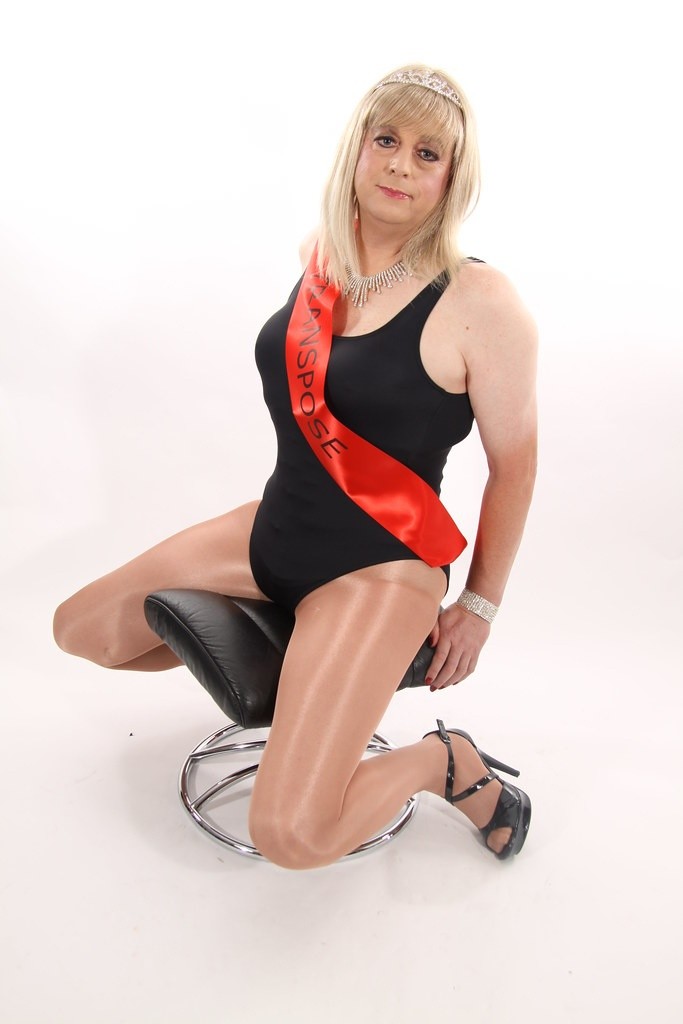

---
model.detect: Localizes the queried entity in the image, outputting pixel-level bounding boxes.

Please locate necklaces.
[341,260,413,307]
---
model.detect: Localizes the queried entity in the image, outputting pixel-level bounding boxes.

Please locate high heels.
[425,718,532,861]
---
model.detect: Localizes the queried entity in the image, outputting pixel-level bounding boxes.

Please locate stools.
[144,589,445,863]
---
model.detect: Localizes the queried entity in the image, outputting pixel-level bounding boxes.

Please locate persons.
[54,65,538,869]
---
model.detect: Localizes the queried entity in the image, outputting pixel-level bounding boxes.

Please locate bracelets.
[456,588,499,624]
